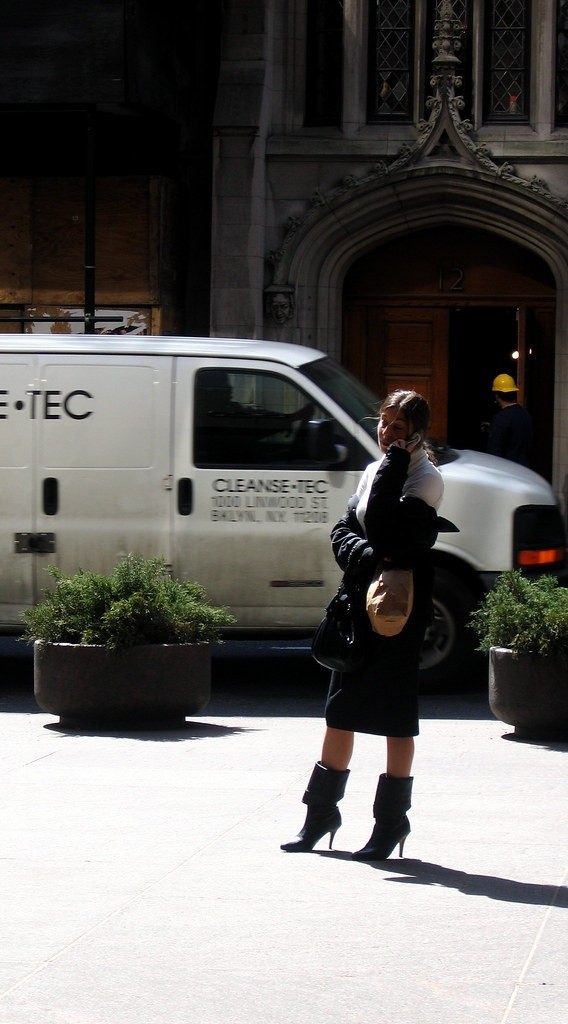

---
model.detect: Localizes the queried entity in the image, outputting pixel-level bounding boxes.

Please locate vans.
[0,335,568,691]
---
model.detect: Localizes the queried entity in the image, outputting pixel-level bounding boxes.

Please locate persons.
[489,373,534,469]
[268,293,290,325]
[281,387,459,862]
[196,369,314,464]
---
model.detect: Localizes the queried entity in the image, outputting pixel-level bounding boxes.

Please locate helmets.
[492,374,520,392]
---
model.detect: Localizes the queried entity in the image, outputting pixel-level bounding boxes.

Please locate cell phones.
[405,432,422,448]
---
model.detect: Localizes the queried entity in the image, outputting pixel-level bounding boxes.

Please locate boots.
[351,773,413,862]
[279,761,350,853]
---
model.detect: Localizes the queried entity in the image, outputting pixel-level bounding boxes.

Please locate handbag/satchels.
[310,538,383,676]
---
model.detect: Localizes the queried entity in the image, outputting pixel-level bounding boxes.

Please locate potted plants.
[14,549,238,732]
[464,568,568,739]
